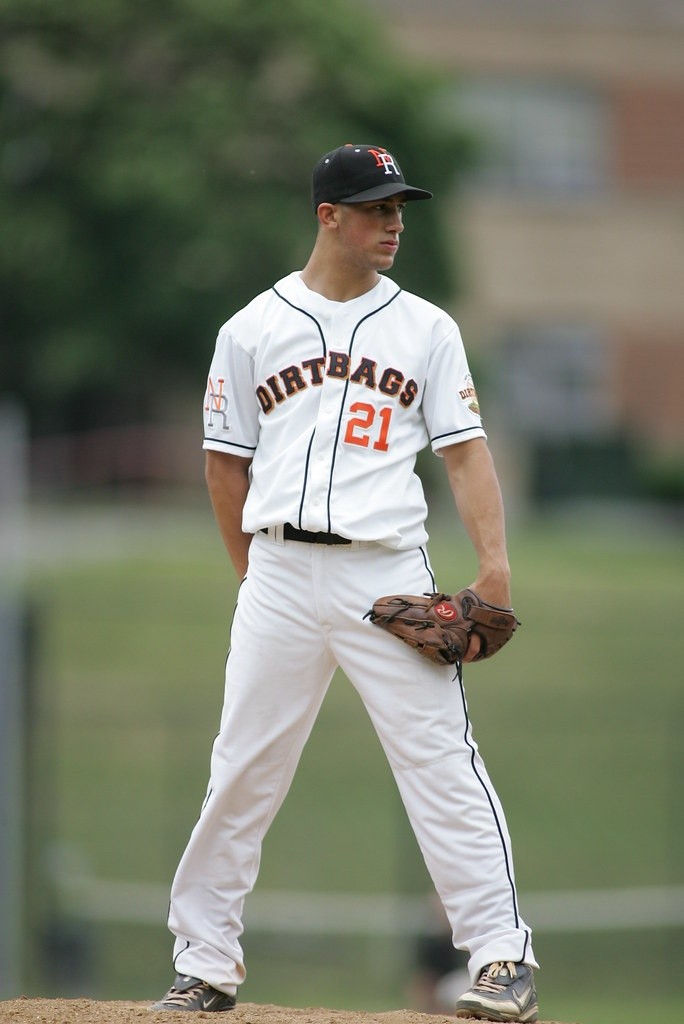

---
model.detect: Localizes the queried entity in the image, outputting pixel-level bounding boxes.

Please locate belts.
[259,524,352,545]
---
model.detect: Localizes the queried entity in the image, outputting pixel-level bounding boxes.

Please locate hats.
[312,144,433,217]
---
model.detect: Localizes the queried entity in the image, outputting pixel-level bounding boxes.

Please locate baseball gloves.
[369,588,518,666]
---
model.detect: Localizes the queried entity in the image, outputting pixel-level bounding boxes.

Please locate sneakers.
[456,961,538,1022]
[147,975,235,1010]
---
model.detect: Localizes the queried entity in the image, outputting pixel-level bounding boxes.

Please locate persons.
[158,140,542,1023]
